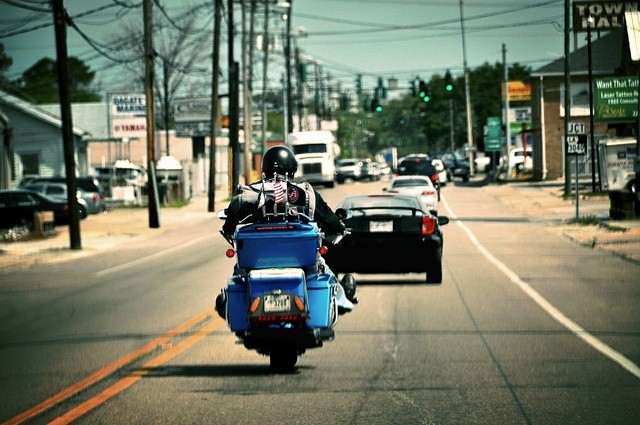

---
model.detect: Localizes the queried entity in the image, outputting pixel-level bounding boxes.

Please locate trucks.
[288,129,336,186]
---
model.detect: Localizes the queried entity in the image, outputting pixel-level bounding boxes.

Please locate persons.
[220,144,346,267]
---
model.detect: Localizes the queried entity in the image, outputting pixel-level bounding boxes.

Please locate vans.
[398,152,440,200]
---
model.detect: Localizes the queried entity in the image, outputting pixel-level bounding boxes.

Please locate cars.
[444,151,469,182]
[432,160,446,186]
[383,175,438,207]
[324,193,449,282]
[339,158,384,180]
[0,188,87,228]
[25,181,105,214]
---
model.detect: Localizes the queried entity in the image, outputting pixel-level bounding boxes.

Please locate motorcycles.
[216,206,358,371]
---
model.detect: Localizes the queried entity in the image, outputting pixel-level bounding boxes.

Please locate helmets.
[261,145,298,176]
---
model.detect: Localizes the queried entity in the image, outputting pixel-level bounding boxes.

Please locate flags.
[286,180,306,207]
[274,181,284,203]
[256,181,266,210]
